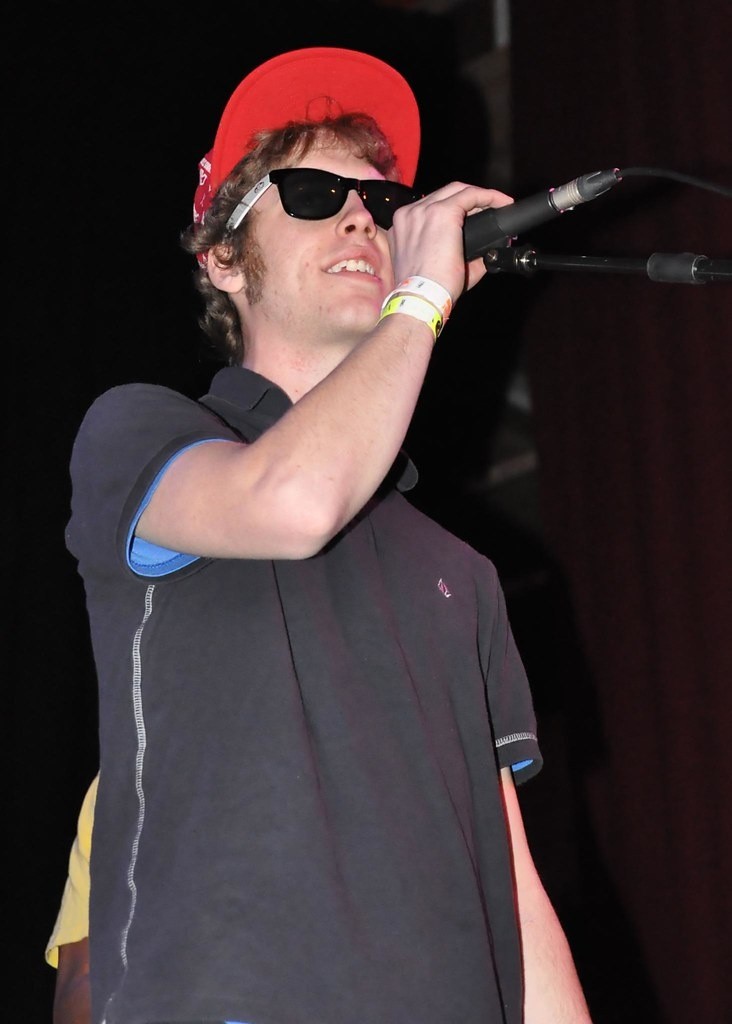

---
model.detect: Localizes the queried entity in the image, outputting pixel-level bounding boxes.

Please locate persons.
[43,768,102,1024]
[66,49,595,1024]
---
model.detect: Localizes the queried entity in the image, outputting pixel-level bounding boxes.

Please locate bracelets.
[379,274,452,337]
[375,295,444,342]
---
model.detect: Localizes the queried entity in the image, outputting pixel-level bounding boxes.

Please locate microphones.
[462,167,621,263]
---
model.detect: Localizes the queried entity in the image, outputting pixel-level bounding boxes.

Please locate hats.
[194,46,421,275]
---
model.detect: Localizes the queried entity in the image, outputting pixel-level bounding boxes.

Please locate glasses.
[223,167,425,244]
